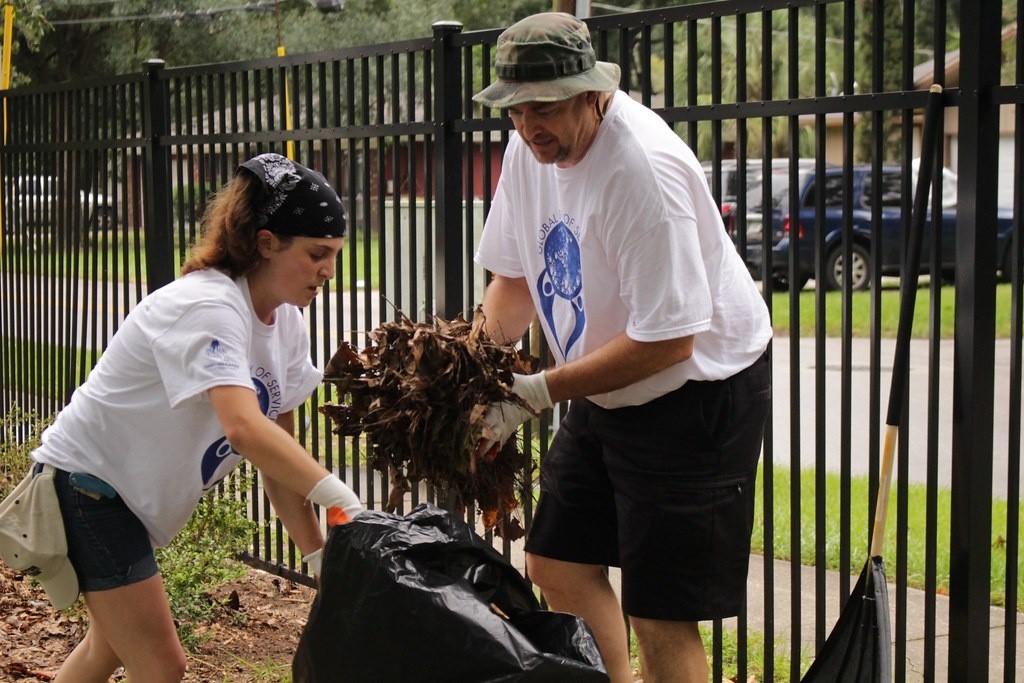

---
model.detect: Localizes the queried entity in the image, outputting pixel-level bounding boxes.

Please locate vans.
[701,159,831,198]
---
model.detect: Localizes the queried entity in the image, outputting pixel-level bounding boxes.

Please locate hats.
[0,461,78,609]
[39,461,39,462]
[472,12,621,108]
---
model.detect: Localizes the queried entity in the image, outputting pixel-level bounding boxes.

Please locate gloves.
[307,474,364,527]
[470,369,555,458]
[302,547,323,584]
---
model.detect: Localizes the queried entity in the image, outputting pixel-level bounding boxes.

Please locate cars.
[719,168,783,233]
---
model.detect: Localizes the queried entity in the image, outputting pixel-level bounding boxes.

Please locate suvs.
[4,177,115,230]
[746,167,1012,291]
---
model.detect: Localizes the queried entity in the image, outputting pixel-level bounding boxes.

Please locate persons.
[28,152,366,683]
[463,13,774,683]
